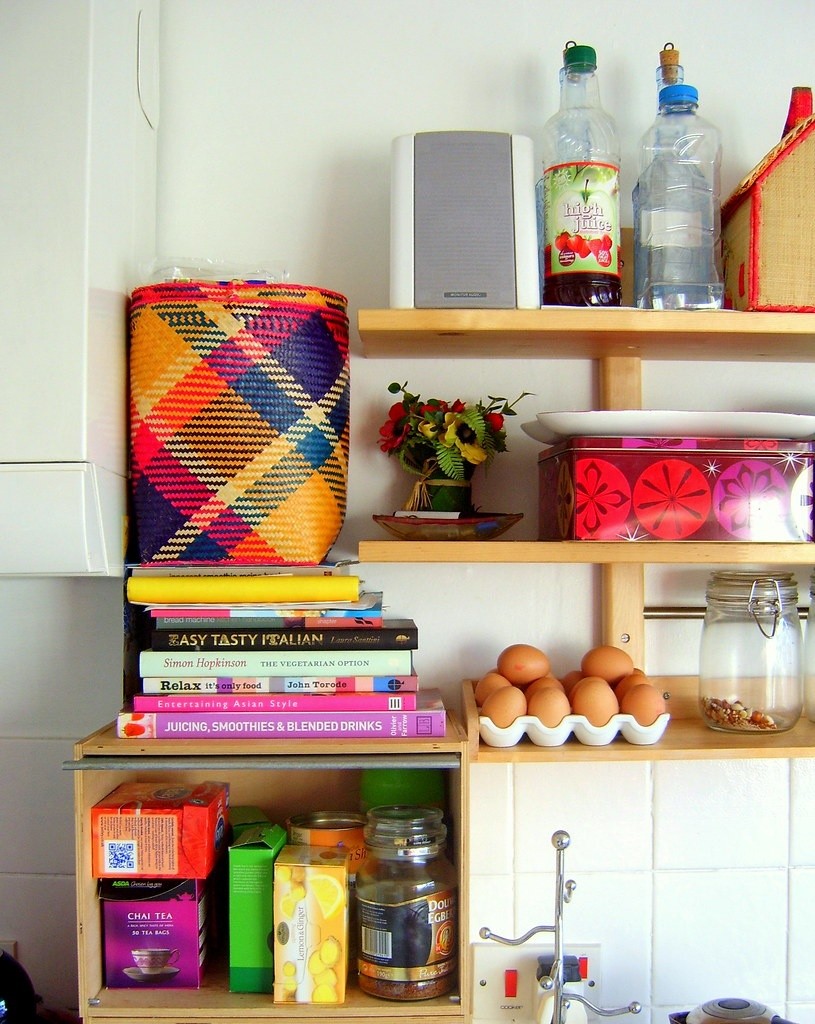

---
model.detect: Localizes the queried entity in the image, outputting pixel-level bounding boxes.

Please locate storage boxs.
[90,780,350,1005]
[537,437,815,544]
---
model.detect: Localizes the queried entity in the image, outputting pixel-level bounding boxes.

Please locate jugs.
[669,1002,792,1024]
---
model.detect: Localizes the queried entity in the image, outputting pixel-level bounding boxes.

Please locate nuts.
[700,697,777,731]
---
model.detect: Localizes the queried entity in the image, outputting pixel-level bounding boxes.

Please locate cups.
[134,947,179,973]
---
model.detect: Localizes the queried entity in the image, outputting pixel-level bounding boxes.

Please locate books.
[117,559,447,740]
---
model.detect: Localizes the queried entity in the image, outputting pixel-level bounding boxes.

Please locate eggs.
[475,644,668,728]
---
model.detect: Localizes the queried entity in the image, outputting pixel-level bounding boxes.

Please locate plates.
[124,968,180,984]
[519,405,815,446]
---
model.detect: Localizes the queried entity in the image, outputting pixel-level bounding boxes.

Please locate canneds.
[284,803,459,1000]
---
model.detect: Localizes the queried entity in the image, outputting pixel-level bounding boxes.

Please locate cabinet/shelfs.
[356,309,815,764]
[71,715,475,1024]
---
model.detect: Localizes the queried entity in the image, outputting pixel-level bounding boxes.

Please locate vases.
[407,444,476,514]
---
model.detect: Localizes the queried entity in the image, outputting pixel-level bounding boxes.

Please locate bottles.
[803,574,814,722]
[352,805,459,1001]
[699,569,802,735]
[533,43,727,310]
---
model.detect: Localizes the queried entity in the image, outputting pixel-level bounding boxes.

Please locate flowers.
[374,379,535,484]
